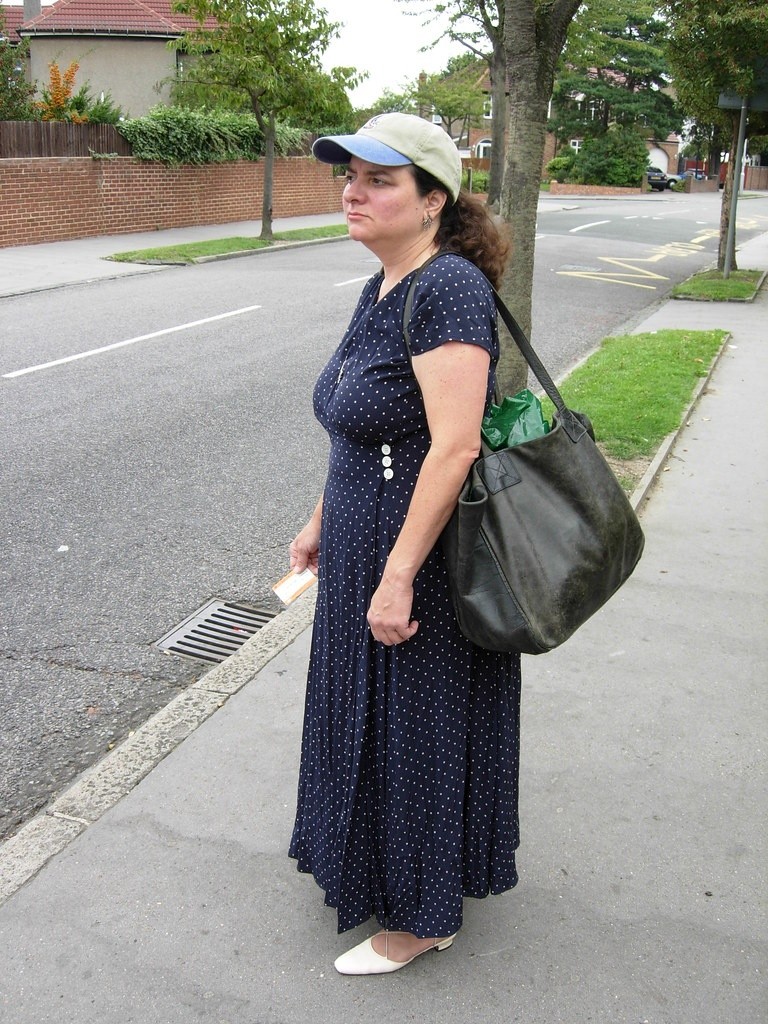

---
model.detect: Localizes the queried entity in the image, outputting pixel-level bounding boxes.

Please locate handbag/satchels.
[403,252,646,655]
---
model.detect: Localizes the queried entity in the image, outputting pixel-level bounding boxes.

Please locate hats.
[312,113,462,206]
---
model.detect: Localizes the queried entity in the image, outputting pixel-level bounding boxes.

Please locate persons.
[288,112,522,975]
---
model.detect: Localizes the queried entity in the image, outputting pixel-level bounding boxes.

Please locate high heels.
[334,928,457,975]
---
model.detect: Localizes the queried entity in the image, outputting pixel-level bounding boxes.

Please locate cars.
[665,174,681,190]
[646,167,667,191]
